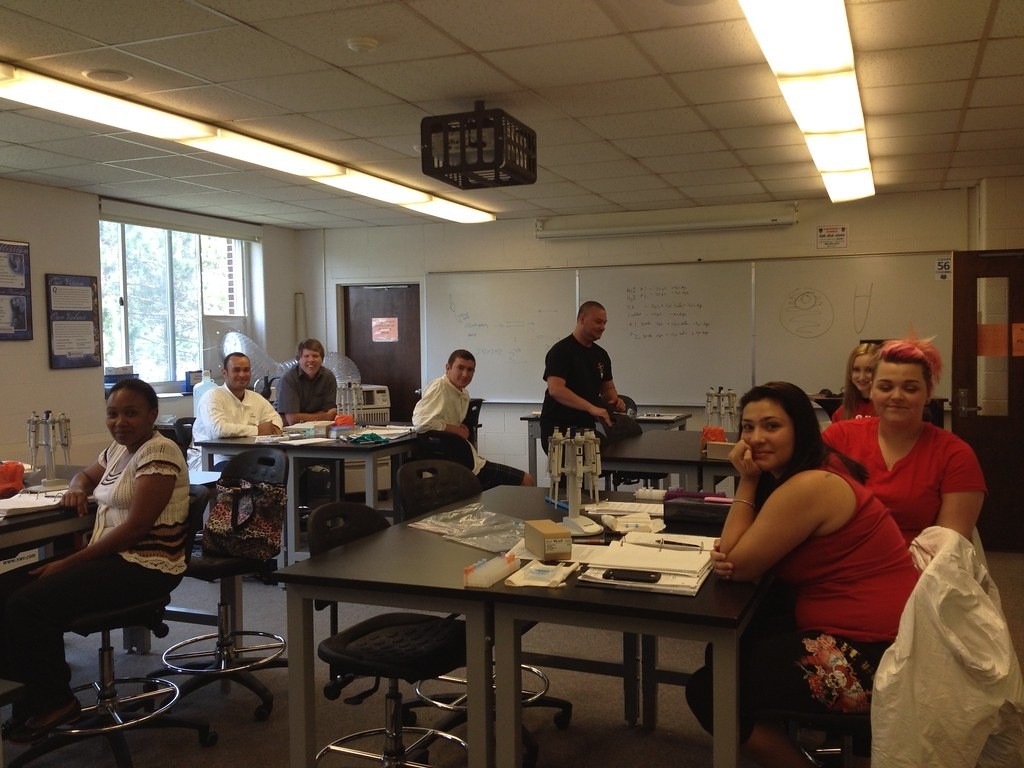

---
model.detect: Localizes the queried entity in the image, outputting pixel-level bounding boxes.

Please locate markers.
[644,414,660,417]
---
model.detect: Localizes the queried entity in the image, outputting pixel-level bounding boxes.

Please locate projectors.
[432,128,525,164]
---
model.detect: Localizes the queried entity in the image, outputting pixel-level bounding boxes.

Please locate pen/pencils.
[656,540,700,548]
[704,496,733,503]
[270,435,284,437]
[574,539,612,545]
[545,496,569,510]
[45,495,63,498]
[362,424,387,427]
[360,441,382,444]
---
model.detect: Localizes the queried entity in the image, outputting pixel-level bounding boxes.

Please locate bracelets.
[732,499,756,509]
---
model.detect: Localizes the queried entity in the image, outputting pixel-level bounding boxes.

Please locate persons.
[824,339,987,547]
[412,349,532,488]
[685,381,918,768]
[539,301,626,487]
[187,352,283,529]
[0,379,190,744]
[272,338,337,426]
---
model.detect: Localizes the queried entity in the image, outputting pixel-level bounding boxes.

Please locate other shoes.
[8,697,82,746]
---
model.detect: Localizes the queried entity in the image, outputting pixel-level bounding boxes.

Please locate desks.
[144,447,290,709]
[808,391,949,430]
[193,421,421,576]
[520,402,692,490]
[0,466,243,661]
[265,484,776,768]
[599,429,740,495]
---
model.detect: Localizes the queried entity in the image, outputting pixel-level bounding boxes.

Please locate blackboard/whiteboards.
[424,250,953,411]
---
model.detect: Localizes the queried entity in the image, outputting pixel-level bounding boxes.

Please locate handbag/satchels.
[202,479,286,560]
[601,414,642,444]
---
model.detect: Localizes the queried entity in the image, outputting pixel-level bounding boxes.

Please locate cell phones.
[705,496,733,504]
[603,568,662,584]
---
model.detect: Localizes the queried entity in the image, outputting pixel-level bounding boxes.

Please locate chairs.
[318,614,571,768]
[46,485,219,768]
